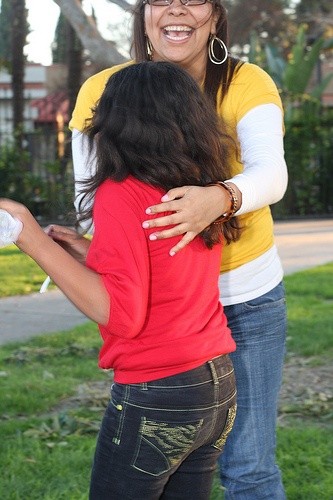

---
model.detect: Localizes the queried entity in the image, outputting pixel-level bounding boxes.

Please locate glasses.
[142,0,211,7]
